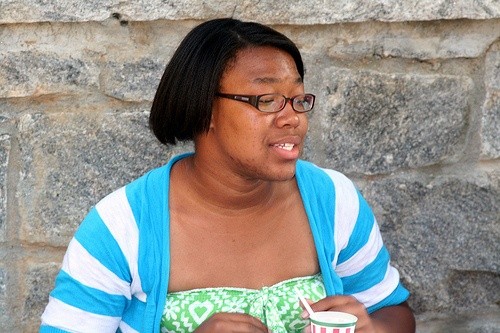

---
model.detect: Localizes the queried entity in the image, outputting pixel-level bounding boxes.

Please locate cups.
[309,311,358,333]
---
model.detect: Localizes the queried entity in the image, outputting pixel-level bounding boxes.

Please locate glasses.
[215,92,316,113]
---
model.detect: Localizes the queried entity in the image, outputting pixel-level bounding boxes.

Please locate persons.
[38,18,416,333]
